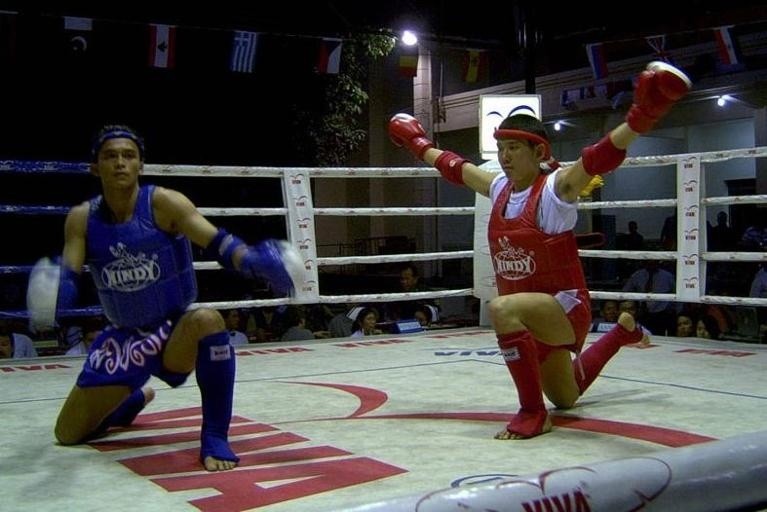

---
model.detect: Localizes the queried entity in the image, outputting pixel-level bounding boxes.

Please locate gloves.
[623,60,693,133]
[239,239,306,298]
[25,258,80,336]
[387,112,434,162]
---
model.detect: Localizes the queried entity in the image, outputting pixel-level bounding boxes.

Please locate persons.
[219,264,458,344]
[25,124,306,472]
[387,56,694,441]
[595,208,767,340]
[1,316,110,358]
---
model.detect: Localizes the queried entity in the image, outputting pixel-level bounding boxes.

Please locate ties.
[645,272,652,293]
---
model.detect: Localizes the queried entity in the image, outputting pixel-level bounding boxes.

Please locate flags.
[458,48,489,85]
[646,36,676,66]
[585,41,608,81]
[227,29,257,74]
[398,45,419,78]
[712,27,745,67]
[146,22,178,70]
[61,17,92,56]
[312,38,343,75]
